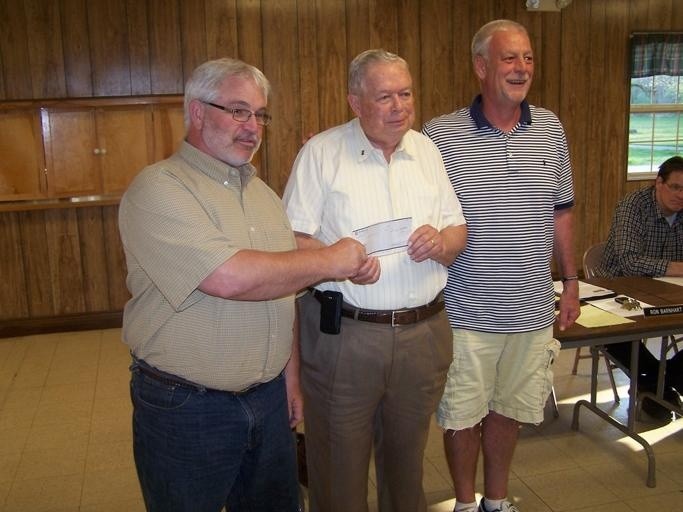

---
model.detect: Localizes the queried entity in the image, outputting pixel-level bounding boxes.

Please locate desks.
[549,276,682,488]
[617,277,682,424]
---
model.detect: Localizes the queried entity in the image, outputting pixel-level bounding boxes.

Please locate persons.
[418,15,583,512]
[589,153,683,419]
[280,48,470,512]
[118,58,366,512]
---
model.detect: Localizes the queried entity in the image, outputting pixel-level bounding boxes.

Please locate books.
[550,280,614,300]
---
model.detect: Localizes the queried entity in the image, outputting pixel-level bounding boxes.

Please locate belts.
[306,287,445,327]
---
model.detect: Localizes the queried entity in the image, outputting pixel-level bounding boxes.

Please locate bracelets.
[560,276,578,280]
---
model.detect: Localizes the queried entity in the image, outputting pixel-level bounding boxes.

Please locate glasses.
[209,102,272,127]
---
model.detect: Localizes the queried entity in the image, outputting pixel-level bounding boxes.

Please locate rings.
[429,239,437,246]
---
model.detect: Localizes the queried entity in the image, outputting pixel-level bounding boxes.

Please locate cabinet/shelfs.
[153,94,256,170]
[0,100,47,213]
[36,95,153,211]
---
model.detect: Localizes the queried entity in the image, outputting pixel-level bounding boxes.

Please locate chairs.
[572,237,680,406]
